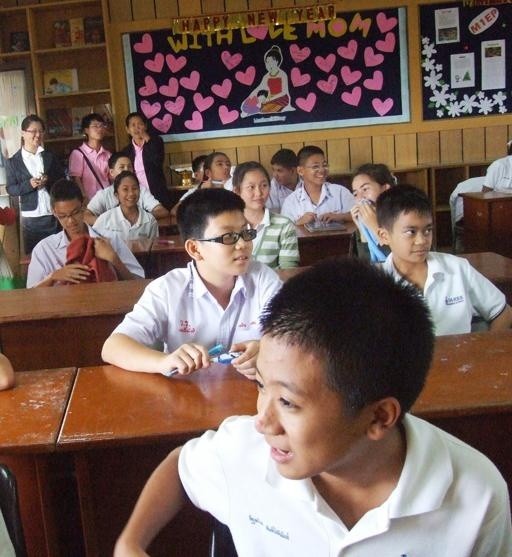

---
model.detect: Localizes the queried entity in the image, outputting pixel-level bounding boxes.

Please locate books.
[8,29,31,52]
[84,15,106,45]
[42,67,80,96]
[69,17,86,47]
[52,20,72,48]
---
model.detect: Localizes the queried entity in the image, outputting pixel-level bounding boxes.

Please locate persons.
[6,113,172,289]
[351,162,395,262]
[375,184,511,337]
[102,358,260,427]
[0,345,18,397]
[113,257,512,557]
[171,146,356,270]
[99,186,289,383]
[480,141,512,194]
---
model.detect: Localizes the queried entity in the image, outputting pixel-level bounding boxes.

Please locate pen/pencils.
[166,344,224,378]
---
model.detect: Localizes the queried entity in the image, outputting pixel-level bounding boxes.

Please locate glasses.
[300,164,329,170]
[191,228,258,246]
[24,128,45,134]
[90,122,107,128]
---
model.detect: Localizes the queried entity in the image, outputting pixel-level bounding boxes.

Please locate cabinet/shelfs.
[0,1,130,159]
[328,163,492,252]
